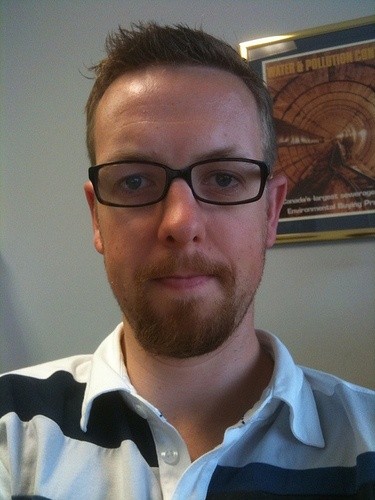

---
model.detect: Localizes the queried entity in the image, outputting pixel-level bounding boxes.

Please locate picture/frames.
[239,15,375,244]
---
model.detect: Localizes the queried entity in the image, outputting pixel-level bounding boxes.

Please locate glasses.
[87,157,274,207]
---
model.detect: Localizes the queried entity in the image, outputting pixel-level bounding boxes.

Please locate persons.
[0,21,375,500]
